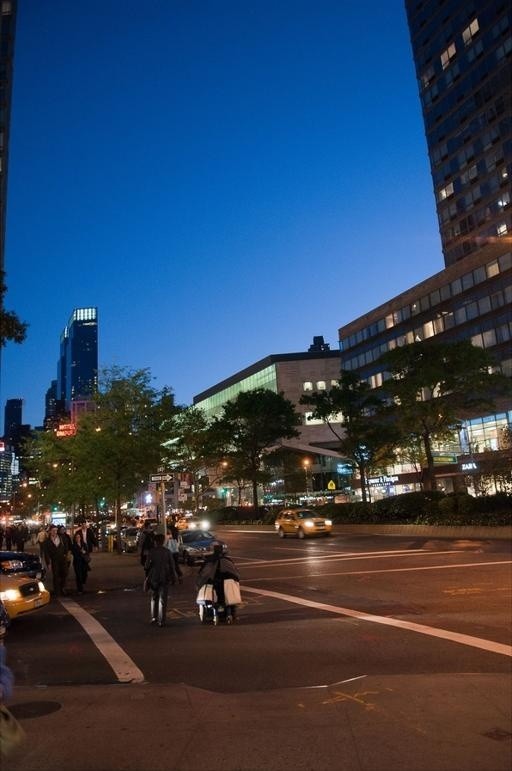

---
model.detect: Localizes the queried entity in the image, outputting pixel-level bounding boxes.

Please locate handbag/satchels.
[80,553,91,563]
[169,559,178,582]
[223,578,242,607]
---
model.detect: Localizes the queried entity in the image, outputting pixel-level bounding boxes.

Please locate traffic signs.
[149,472,175,482]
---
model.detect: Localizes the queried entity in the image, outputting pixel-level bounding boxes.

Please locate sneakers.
[148,618,164,628]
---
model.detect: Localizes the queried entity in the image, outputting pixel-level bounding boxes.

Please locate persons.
[0,600,14,720]
[165,525,184,585]
[198,544,234,572]
[144,533,181,628]
[138,521,156,564]
[0,518,99,596]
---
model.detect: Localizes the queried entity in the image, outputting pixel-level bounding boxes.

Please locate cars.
[0,573,50,619]
[274,508,334,538]
[91,516,229,566]
[0,549,47,581]
